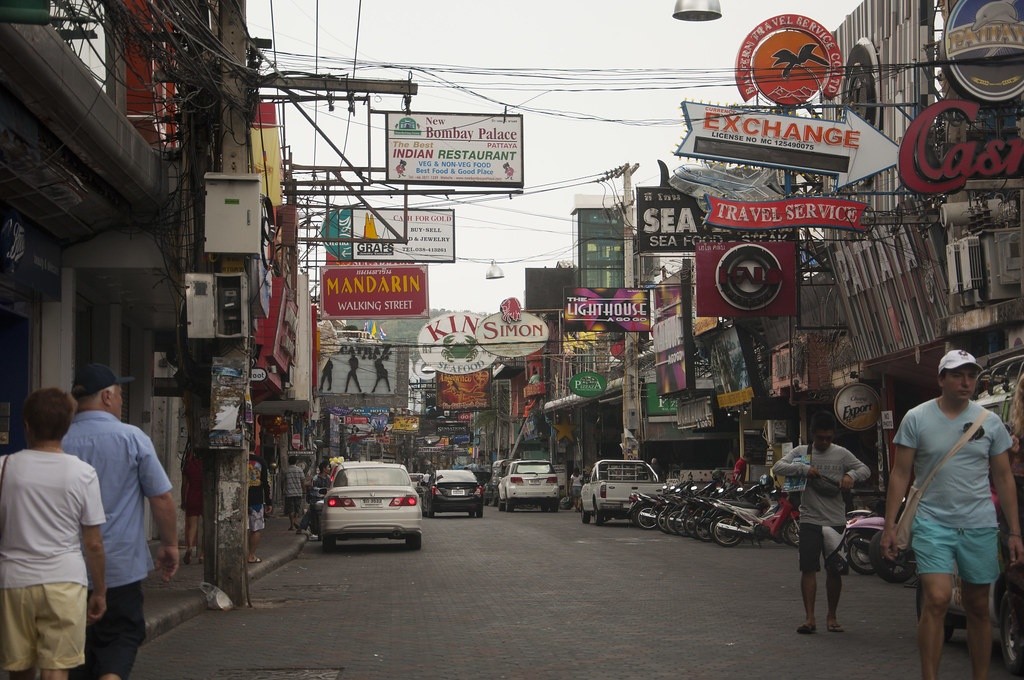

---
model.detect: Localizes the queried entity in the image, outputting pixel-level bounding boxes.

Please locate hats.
[71,363,135,399]
[938,349,983,376]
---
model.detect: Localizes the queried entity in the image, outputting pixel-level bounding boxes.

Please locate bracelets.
[1009,533,1023,538]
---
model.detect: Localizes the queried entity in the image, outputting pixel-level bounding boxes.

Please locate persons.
[0,389,108,680]
[880,350,1024,680]
[178,434,330,568]
[772,412,872,633]
[732,450,753,486]
[993,373,1024,538]
[57,363,180,680]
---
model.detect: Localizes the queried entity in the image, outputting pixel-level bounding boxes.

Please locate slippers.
[797,623,816,633]
[827,625,843,632]
[247,558,262,563]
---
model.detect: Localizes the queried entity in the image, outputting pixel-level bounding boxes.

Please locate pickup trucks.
[580,459,667,526]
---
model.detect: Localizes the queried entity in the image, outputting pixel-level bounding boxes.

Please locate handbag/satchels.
[807,474,841,498]
[895,485,921,550]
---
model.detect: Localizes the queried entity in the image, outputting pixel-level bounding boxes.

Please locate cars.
[311,458,560,552]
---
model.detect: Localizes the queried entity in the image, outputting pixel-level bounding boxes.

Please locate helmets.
[760,473,774,489]
[711,469,723,480]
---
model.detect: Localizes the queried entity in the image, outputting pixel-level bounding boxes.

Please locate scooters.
[627,468,885,575]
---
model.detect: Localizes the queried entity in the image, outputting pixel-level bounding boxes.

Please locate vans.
[914,354,1024,680]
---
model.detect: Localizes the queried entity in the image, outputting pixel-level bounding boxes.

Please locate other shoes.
[183,548,192,564]
[296,529,302,534]
[199,557,204,564]
